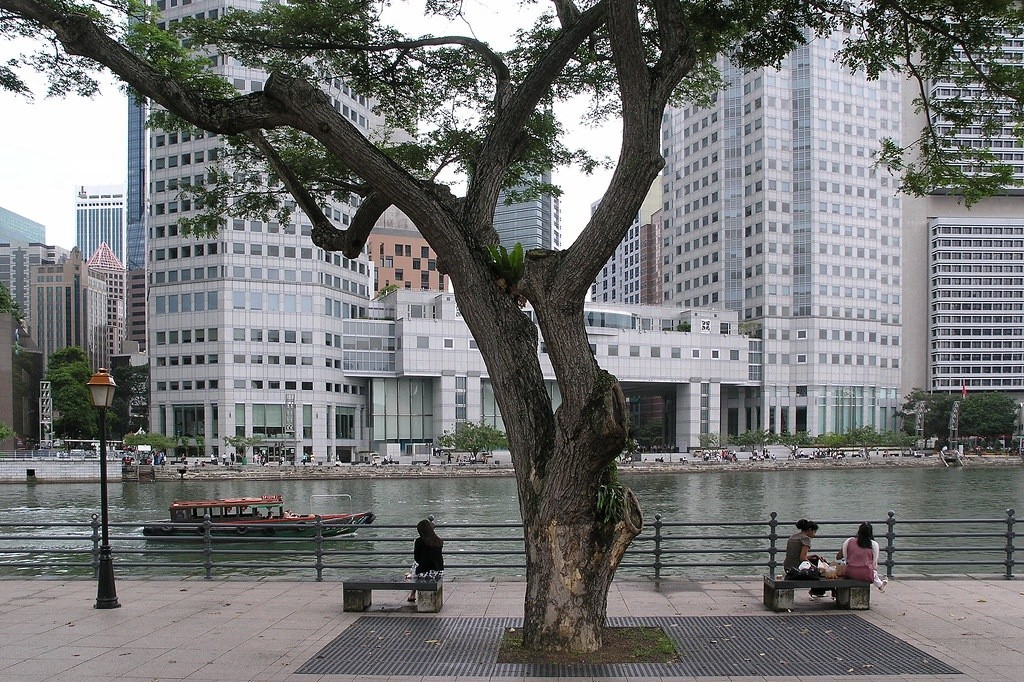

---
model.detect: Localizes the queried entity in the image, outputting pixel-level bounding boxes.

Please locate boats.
[144,495,376,537]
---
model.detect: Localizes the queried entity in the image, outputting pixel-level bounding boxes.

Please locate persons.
[222,453,227,465]
[311,453,316,465]
[383,455,393,463]
[470,455,475,464]
[211,452,215,464]
[252,452,266,466]
[843,521,888,590]
[407,520,444,601]
[181,453,186,461]
[784,519,836,600]
[336,454,339,461]
[257,509,301,520]
[682,445,889,464]
[279,457,284,465]
[481,455,487,464]
[114,444,167,465]
[303,453,308,465]
[231,454,235,465]
[448,451,452,463]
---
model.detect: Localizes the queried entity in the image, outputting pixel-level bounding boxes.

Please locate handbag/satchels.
[785,567,822,581]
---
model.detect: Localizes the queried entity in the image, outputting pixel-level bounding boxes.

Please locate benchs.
[342,574,444,612]
[763,574,870,611]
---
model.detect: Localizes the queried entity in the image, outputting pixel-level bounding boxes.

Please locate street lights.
[426,441,436,467]
[87,368,122,608]
[667,444,675,466]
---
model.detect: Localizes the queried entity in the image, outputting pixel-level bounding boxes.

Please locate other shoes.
[406,596,416,603]
[878,580,888,590]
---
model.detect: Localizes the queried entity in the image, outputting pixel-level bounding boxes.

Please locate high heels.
[808,589,826,598]
